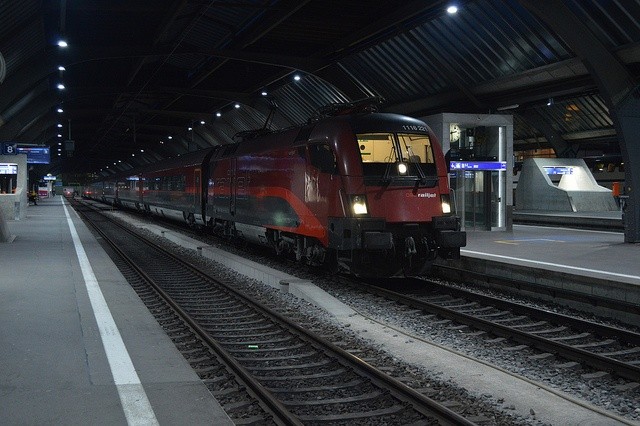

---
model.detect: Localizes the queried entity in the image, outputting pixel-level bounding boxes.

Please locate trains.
[79,95,466,281]
